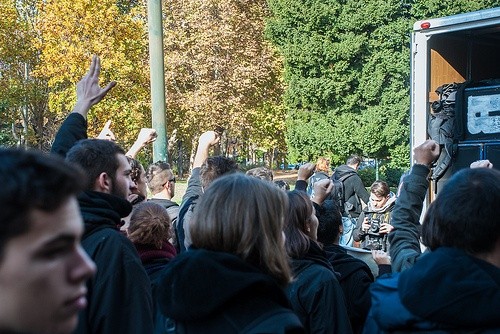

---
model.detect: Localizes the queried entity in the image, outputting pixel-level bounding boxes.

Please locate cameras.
[367,219,384,237]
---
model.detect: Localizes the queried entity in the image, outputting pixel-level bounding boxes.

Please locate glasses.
[162,176,176,187]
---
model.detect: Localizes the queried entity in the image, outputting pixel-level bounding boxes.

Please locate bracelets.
[417,163,429,167]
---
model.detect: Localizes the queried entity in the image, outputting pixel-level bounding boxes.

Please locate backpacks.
[334,171,357,214]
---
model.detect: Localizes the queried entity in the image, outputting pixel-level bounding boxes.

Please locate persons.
[0,54,500,334]
[331,155,369,246]
[353,180,397,251]
